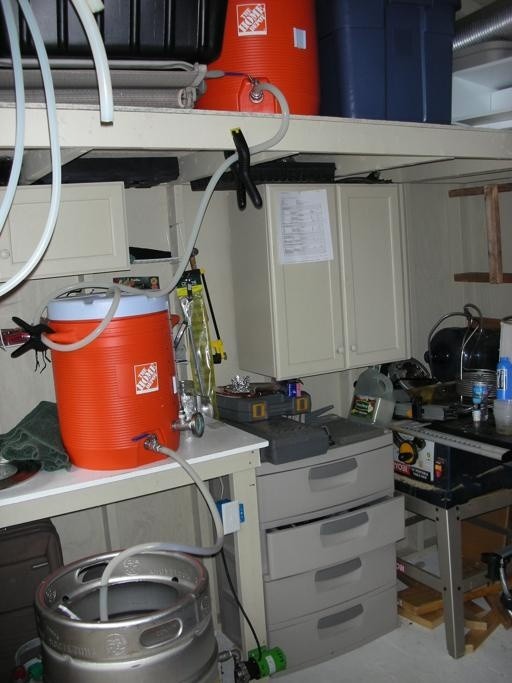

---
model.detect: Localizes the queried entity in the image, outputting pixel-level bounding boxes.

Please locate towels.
[0,400,72,472]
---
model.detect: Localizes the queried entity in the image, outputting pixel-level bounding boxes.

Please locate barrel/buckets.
[348,367,397,425]
[35,548,221,683]
[47,294,179,470]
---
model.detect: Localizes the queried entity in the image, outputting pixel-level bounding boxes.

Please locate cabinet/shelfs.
[209,415,406,679]
[0,182,130,283]
[228,182,411,383]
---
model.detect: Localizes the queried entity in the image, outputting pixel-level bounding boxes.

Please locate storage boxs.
[315,0,461,126]
[0,0,228,64]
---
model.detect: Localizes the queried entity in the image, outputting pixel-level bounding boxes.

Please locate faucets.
[132,433,160,453]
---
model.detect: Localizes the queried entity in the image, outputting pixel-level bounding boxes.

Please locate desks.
[377,421,511,662]
[0,416,269,662]
